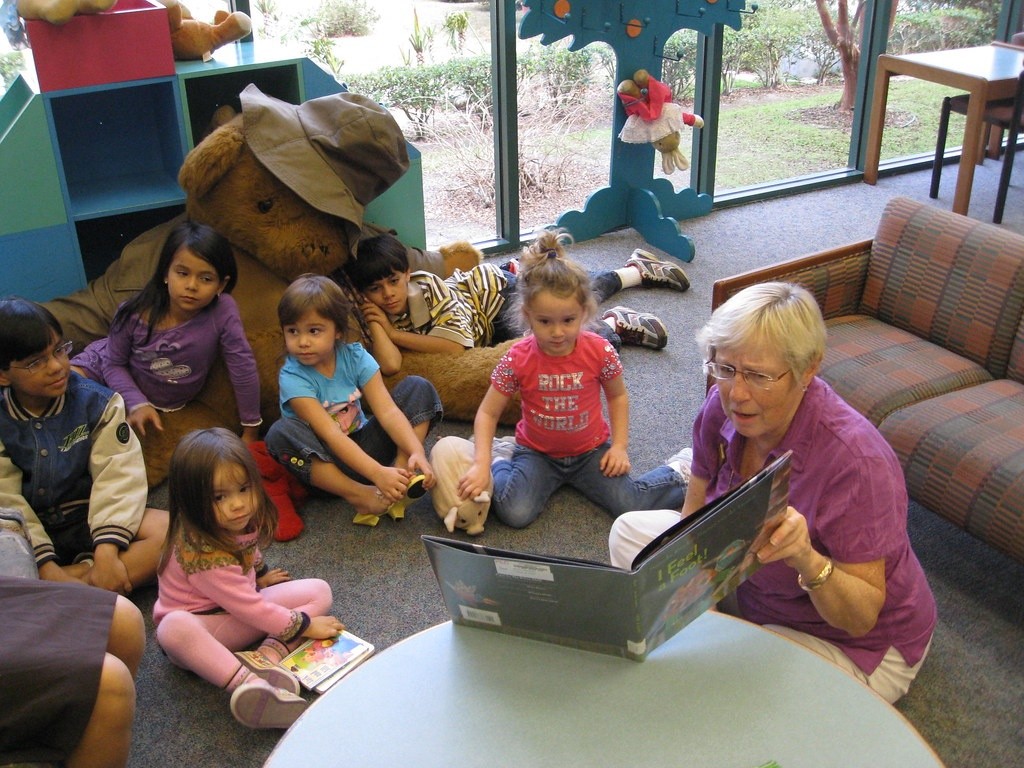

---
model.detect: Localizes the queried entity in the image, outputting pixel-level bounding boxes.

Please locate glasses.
[702,354,788,390]
[0,338,73,375]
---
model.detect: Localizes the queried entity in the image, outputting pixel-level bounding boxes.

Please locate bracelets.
[798,556,834,592]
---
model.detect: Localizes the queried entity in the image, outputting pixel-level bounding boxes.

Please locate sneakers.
[624,249,688,294]
[669,448,696,475]
[469,433,517,461]
[602,304,667,349]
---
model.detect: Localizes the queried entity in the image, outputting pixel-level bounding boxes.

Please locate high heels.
[386,472,431,523]
[352,498,399,527]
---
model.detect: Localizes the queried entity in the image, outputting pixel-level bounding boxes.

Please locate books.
[277,627,375,695]
[418,447,797,663]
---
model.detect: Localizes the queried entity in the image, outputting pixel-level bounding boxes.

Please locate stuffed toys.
[0,0,252,61]
[613,69,705,176]
[36,83,535,482]
[430,435,493,536]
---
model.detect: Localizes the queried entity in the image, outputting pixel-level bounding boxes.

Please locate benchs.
[705,197,1023,562]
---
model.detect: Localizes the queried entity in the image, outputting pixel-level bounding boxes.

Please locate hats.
[238,82,415,240]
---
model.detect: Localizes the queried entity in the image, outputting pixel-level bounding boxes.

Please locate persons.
[456,226,693,528]
[0,572,147,768]
[153,426,345,732]
[605,282,935,708]
[66,221,263,442]
[0,295,169,595]
[351,234,690,375]
[265,272,444,527]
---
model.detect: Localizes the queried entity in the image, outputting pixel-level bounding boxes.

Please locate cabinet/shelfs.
[0,1,426,299]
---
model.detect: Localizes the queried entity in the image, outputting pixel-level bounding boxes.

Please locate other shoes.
[235,650,301,697]
[231,681,306,729]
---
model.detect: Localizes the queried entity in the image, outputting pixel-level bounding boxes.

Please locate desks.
[863,42,1024,216]
[264,611,948,768]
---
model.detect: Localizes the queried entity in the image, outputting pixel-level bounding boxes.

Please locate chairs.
[929,59,1024,224]
